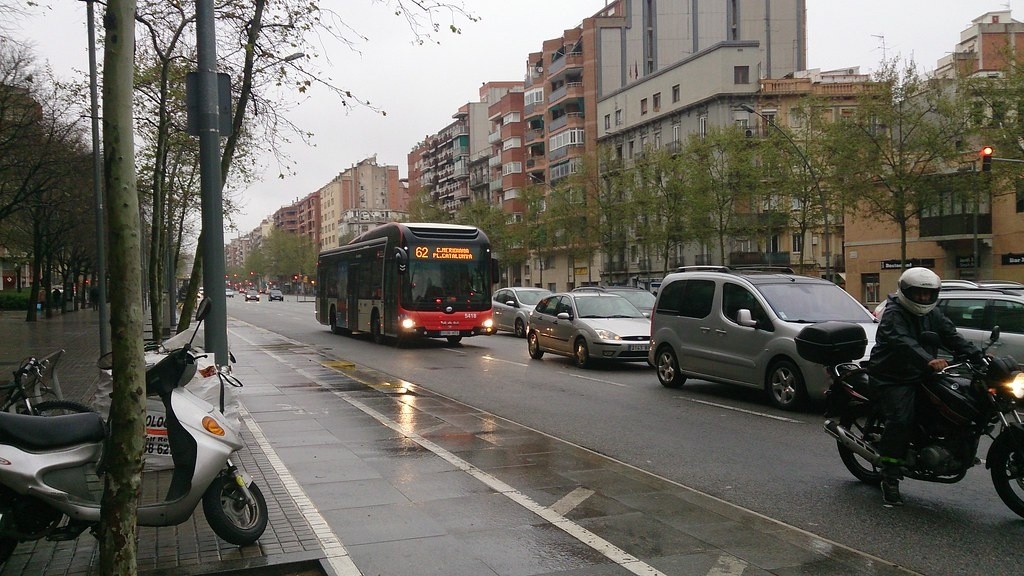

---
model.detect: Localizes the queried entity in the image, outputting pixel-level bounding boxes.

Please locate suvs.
[645,265,883,413]
[872,279,1024,370]
[569,285,656,321]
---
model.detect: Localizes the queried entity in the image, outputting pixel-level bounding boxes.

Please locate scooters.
[0,292,270,563]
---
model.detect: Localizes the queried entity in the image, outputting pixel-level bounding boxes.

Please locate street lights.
[740,103,832,281]
[523,170,592,285]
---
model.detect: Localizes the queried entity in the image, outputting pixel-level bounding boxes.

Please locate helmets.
[896,267,941,316]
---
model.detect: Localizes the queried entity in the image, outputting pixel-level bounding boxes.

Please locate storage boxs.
[794,321,868,366]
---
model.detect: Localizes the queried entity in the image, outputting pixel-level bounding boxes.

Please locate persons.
[54,288,61,313]
[457,278,472,295]
[90,284,99,311]
[869,268,995,508]
[40,290,45,313]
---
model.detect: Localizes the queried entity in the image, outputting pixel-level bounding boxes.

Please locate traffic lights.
[982,147,993,173]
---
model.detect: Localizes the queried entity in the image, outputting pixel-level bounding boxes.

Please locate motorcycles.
[822,325,1024,520]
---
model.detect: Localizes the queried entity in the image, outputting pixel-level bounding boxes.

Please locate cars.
[491,287,555,338]
[199,287,204,295]
[245,290,260,301]
[226,288,234,298]
[239,288,245,294]
[527,292,652,368]
[268,290,283,301]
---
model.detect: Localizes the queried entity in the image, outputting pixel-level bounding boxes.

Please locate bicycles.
[0,354,95,417]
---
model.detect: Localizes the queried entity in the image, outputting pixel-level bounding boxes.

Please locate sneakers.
[973,457,980,464]
[881,480,904,505]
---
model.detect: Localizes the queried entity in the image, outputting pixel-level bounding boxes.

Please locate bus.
[314,221,500,347]
